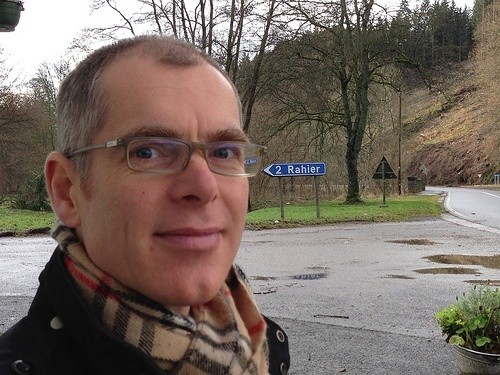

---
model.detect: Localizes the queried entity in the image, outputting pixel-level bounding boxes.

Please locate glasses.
[66,136,268,178]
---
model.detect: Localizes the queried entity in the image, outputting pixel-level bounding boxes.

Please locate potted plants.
[436,279,500,375]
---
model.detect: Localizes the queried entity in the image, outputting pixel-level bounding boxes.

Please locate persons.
[0,34,290,375]
